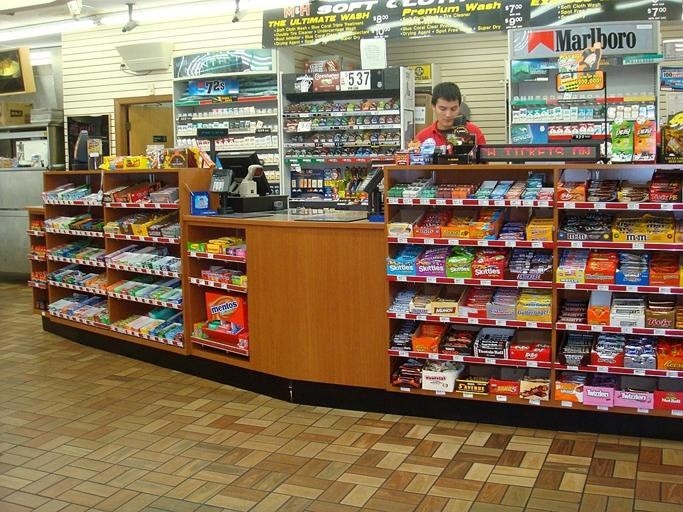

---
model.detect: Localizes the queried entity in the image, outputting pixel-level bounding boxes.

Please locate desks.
[183,210,387,391]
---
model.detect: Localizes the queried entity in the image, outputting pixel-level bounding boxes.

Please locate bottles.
[338,166,367,198]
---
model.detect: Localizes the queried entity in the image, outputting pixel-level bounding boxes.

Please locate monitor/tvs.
[217,152,273,195]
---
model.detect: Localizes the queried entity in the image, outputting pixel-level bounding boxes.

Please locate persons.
[411,82,486,147]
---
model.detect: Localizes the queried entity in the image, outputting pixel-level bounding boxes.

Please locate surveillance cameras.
[232,11,245,22]
[121,21,138,32]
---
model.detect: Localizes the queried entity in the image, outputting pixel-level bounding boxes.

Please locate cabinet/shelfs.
[382,164,683,420]
[279,67,415,213]
[23,168,219,356]
[507,18,662,164]
[170,45,295,196]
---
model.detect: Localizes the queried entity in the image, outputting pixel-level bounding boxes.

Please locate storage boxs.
[413,94,432,136]
